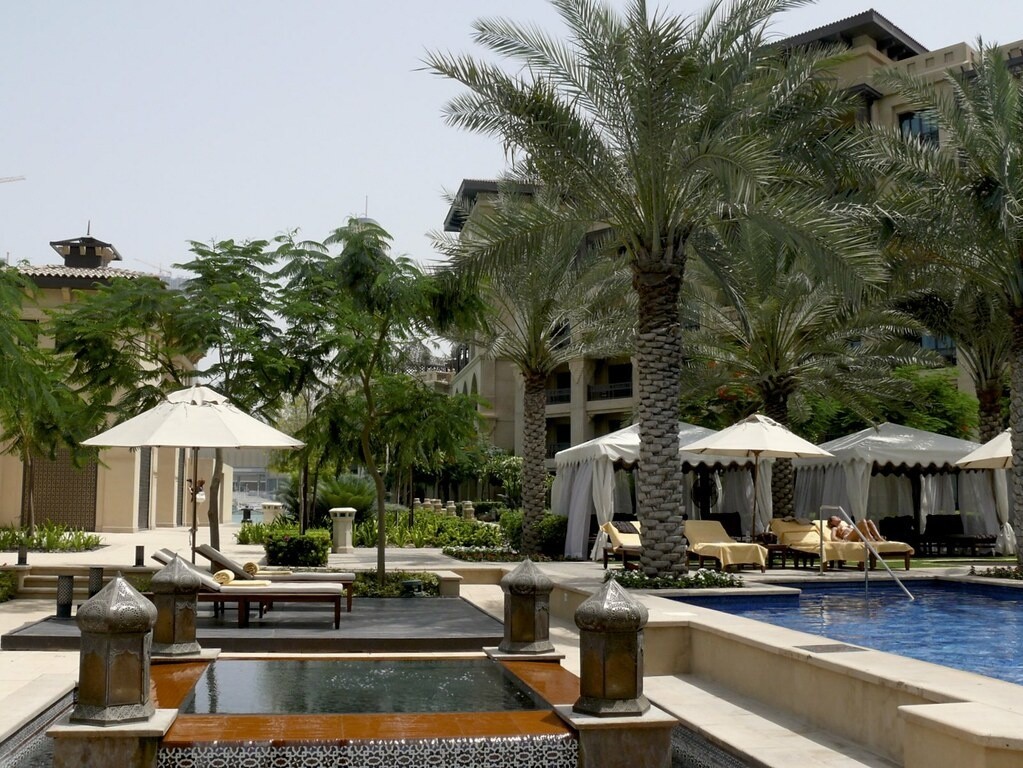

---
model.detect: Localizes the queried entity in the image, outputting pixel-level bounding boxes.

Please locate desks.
[761,544,789,568]
[213,601,267,618]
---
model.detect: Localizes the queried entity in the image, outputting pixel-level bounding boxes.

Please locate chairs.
[589,513,637,547]
[879,515,929,558]
[812,519,914,570]
[683,520,768,573]
[191,545,356,613]
[599,521,688,570]
[707,513,746,543]
[150,547,343,630]
[923,512,998,558]
[767,517,870,572]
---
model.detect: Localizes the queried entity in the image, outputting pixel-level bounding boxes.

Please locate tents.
[551,421,776,559]
[793,423,1016,556]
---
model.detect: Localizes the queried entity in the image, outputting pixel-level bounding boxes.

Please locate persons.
[828,515,886,543]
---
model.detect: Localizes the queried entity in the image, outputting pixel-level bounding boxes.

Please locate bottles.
[746,529,750,542]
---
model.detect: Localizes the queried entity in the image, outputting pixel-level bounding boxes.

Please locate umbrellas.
[679,410,836,541]
[79,384,307,565]
[955,426,1013,469]
